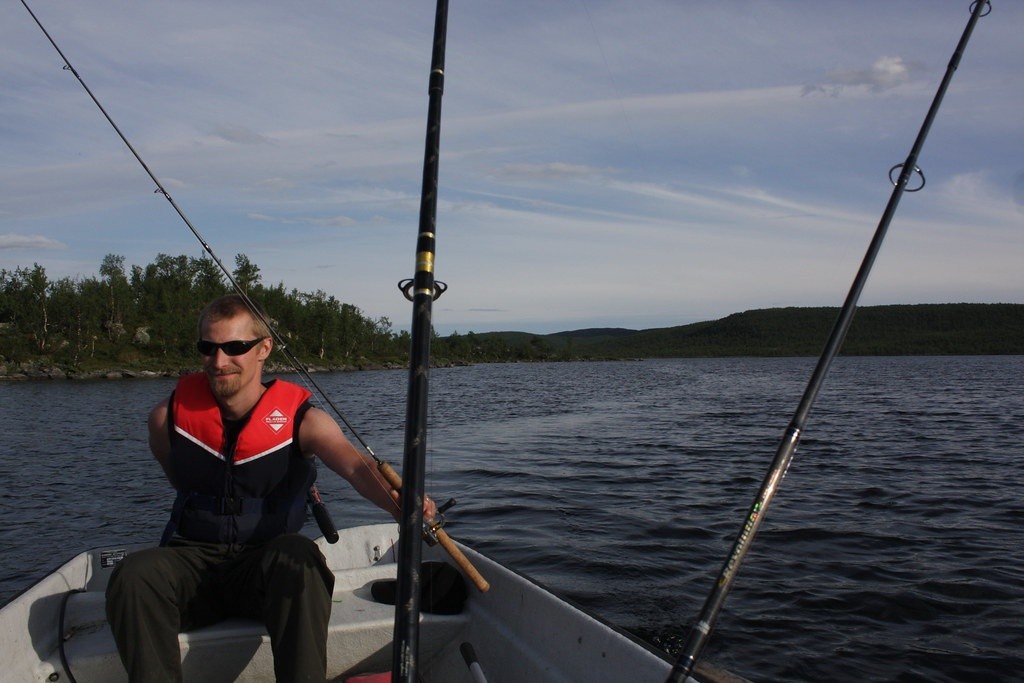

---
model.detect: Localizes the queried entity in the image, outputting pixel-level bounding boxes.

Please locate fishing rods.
[391,0,452,683]
[20,0,494,595]
[664,0,992,683]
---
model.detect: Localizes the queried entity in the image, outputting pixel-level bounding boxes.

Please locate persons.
[104,294,438,683]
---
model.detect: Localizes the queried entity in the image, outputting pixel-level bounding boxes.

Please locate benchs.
[58,563,471,683]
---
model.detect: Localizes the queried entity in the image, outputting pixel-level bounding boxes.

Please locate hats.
[371,561,467,615]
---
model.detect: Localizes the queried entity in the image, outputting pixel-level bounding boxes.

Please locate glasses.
[197,337,265,356]
